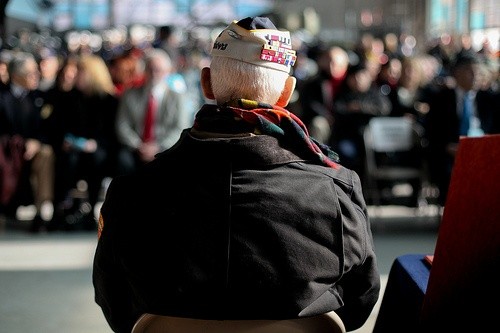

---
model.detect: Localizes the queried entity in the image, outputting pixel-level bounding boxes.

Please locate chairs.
[371,134,500,333]
[363,116,429,208]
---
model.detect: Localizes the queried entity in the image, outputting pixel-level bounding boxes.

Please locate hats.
[211,16,296,73]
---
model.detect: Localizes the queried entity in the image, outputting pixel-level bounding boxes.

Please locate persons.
[92,17,381,333]
[0,23,210,230]
[289,25,500,209]
